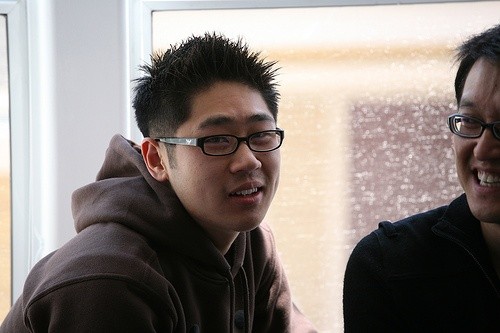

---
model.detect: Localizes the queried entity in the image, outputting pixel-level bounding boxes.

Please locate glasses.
[151,127,285,156]
[446,114,500,141]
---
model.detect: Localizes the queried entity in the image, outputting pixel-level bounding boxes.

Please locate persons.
[0,34,322,333]
[342,25,500,333]
